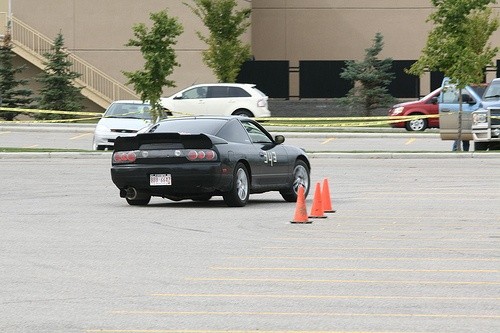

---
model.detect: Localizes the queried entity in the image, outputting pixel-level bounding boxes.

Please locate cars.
[93,98,161,152]
[389,83,490,131]
[159,82,272,123]
[438,76,500,149]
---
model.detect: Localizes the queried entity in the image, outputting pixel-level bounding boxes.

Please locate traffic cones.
[289,184,312,224]
[321,178,336,213]
[308,182,329,219]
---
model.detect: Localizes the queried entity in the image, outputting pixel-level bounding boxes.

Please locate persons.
[452,88,476,152]
[142,107,151,118]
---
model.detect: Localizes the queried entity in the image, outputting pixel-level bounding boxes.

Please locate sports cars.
[110,115,311,207]
